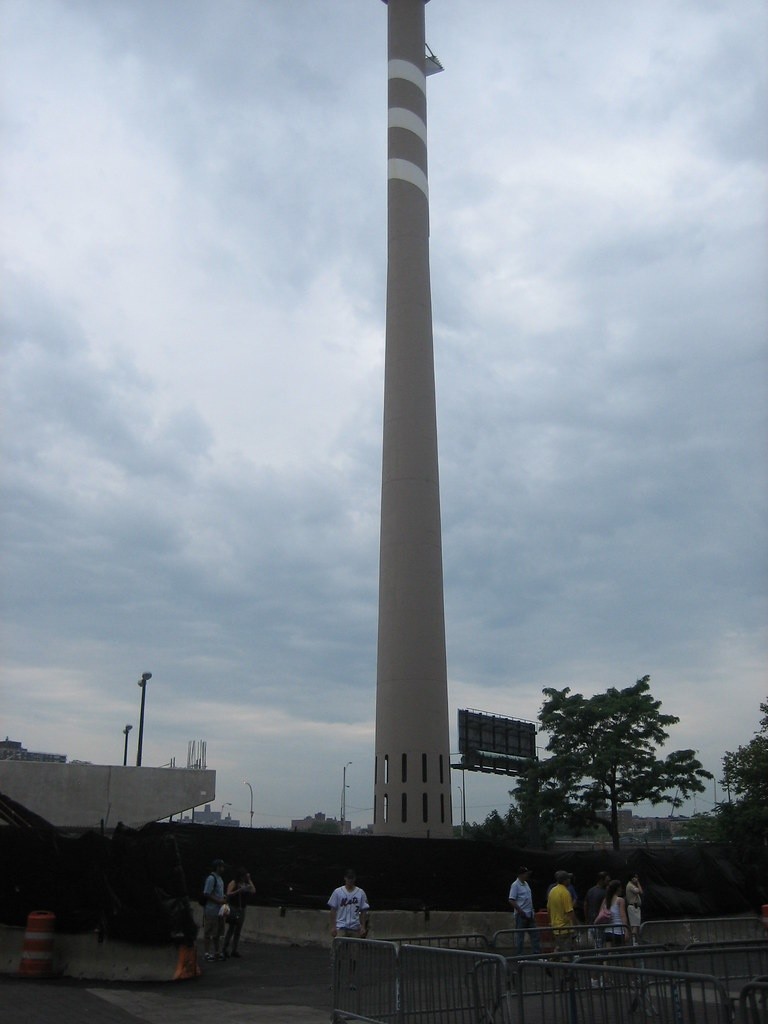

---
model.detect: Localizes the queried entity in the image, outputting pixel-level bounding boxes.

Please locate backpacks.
[198,874,223,906]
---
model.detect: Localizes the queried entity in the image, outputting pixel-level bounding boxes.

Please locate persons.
[204,859,227,962]
[509,866,547,963]
[222,869,257,959]
[327,867,370,991]
[544,871,644,988]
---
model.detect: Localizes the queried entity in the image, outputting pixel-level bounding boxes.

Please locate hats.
[518,865,532,874]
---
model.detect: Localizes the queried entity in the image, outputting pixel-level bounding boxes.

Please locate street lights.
[122,725,132,766]
[244,781,254,827]
[137,672,152,766]
[341,762,352,835]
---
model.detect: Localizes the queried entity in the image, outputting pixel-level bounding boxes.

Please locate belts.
[629,903,641,907]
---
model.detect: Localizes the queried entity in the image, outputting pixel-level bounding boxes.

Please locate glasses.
[217,859,224,865]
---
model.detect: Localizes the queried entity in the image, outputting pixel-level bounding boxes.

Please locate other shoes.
[231,950,242,957]
[328,984,334,991]
[590,977,600,986]
[631,977,640,986]
[539,958,547,963]
[214,951,224,961]
[517,959,527,963]
[349,983,357,991]
[633,943,639,946]
[205,954,215,962]
[223,950,230,958]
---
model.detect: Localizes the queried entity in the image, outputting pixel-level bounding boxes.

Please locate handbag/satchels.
[594,904,612,925]
[218,902,230,917]
[225,905,242,924]
